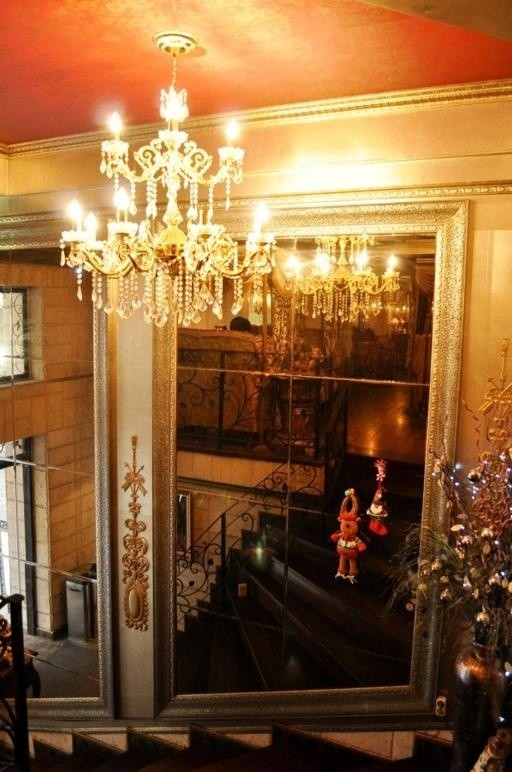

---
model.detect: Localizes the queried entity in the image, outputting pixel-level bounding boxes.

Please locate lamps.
[279,233,410,326]
[57,31,276,328]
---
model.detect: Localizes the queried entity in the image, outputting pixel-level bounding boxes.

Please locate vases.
[450,637,504,766]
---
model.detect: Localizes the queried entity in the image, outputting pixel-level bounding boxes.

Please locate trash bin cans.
[66,571,98,643]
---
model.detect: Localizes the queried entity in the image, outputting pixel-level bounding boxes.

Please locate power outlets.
[435,695,448,718]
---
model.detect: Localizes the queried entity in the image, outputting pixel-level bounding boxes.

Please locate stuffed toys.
[329,485,366,586]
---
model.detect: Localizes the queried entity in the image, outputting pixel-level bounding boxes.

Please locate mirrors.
[148,190,478,727]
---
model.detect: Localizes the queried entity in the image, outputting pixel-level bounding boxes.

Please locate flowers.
[384,337,512,663]
